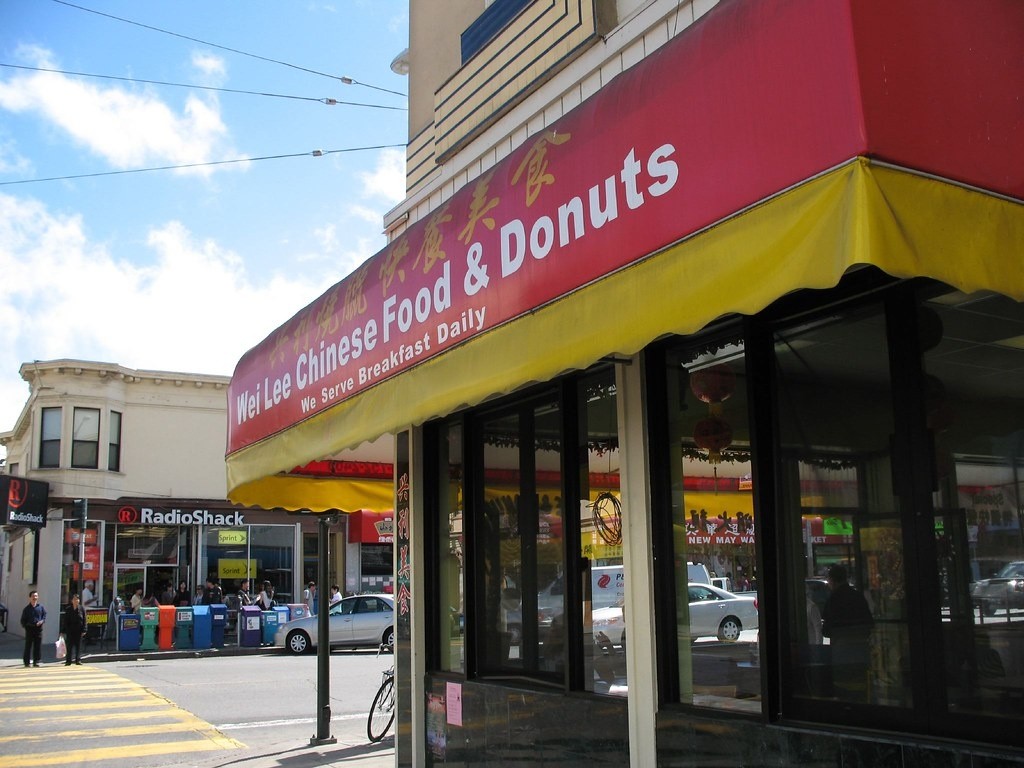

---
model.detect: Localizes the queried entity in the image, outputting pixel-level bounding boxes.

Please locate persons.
[130,575,276,614]
[330,584,343,615]
[20,591,47,667]
[806,596,822,647]
[303,581,316,616]
[821,563,877,640]
[59,593,87,666]
[82,580,99,606]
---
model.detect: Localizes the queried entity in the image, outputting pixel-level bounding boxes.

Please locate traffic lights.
[71,500,87,529]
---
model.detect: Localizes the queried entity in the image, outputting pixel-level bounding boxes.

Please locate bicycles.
[368,643,398,743]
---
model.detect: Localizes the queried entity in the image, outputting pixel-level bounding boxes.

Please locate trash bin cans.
[0,603,9,633]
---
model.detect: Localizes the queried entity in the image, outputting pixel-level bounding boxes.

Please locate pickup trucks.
[710,578,757,598]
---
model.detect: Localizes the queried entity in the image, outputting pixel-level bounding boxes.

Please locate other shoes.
[86,640,97,646]
[65,663,71,666]
[33,662,39,667]
[76,663,82,665]
[25,664,31,667]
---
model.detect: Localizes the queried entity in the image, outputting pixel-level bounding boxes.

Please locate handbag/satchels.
[55,636,67,658]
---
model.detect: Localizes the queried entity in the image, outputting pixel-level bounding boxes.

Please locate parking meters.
[236,594,243,648]
[114,596,124,652]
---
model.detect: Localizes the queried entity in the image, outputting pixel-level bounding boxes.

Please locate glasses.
[196,588,201,590]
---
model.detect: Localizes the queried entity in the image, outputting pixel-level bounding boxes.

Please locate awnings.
[225,1,1023,520]
[348,510,394,543]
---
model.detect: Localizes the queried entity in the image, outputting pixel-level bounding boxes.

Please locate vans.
[538,562,713,618]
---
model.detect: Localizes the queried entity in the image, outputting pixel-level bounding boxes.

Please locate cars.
[458,606,553,646]
[224,595,291,633]
[274,594,397,651]
[591,581,758,651]
[972,561,1024,616]
